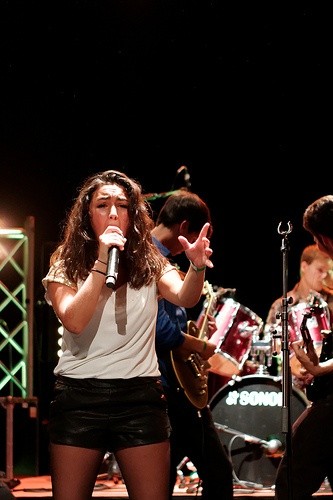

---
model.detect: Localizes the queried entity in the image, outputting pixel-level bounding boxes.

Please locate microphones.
[267,436,282,453]
[105,231,121,288]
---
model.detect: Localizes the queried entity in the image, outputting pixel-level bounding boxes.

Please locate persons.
[275,195,333,496]
[262,245,331,493]
[151,191,224,498]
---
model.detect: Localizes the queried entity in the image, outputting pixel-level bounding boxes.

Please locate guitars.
[170,286,236,412]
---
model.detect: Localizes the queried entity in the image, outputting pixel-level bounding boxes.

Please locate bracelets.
[43,169,214,500]
[97,259,108,266]
[188,258,206,272]
[198,341,207,355]
[90,269,107,277]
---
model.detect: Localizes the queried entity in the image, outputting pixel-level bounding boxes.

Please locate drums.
[191,296,264,377]
[205,374,310,490]
[271,303,332,378]
[220,361,266,386]
